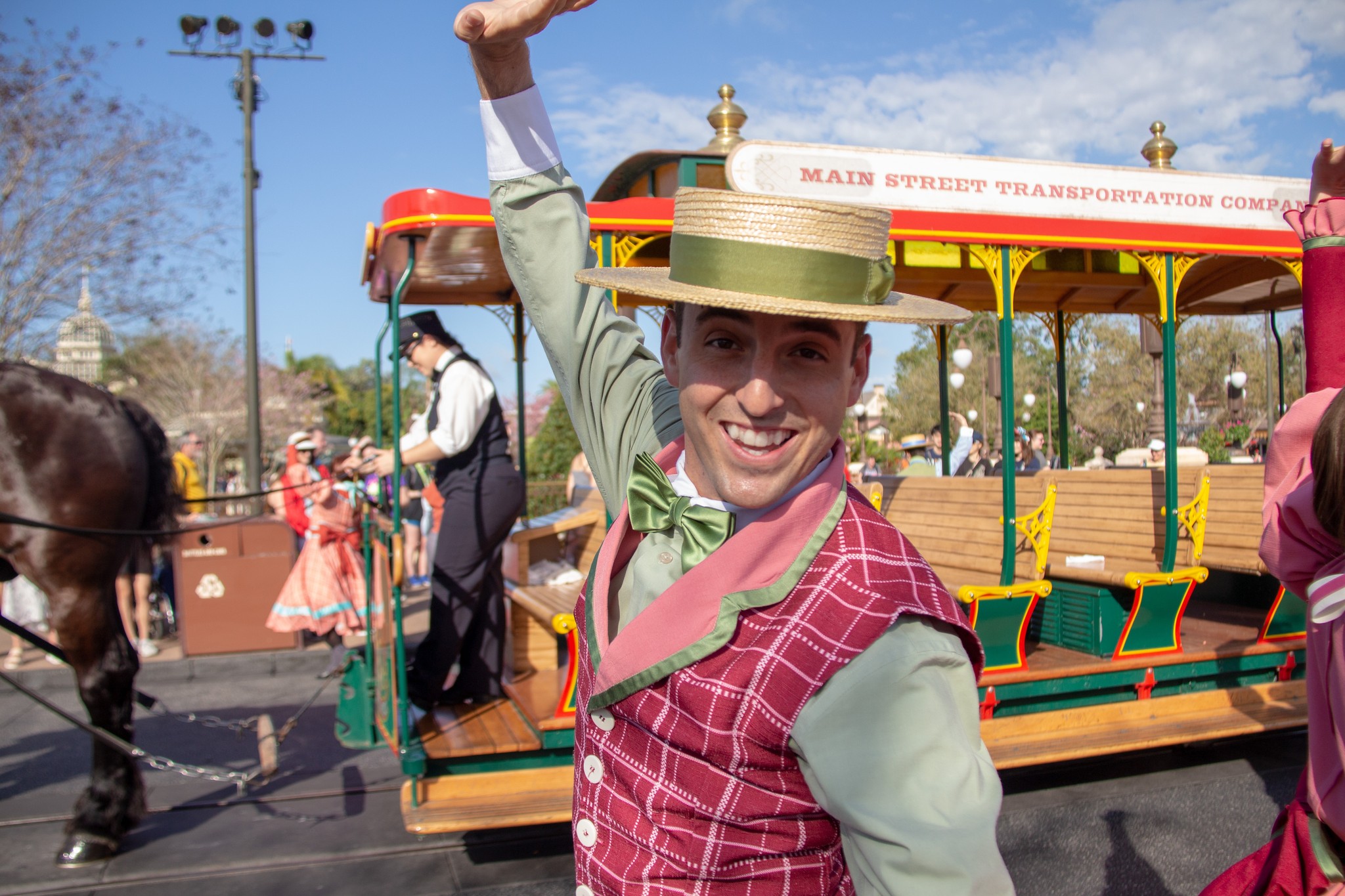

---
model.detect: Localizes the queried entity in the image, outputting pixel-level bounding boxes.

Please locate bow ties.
[626,451,737,577]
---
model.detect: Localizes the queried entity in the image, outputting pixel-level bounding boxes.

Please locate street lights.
[164,13,328,513]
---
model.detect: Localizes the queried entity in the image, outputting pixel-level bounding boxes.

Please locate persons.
[1196,381,1345,895]
[114,413,457,659]
[561,445,599,570]
[449,0,1024,896]
[353,304,524,710]
[0,573,67,671]
[839,363,1298,479]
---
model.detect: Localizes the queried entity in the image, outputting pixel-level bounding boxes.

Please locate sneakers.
[407,575,431,588]
[319,642,351,678]
[128,636,159,657]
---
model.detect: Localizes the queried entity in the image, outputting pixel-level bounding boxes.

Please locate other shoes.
[44,652,64,665]
[391,585,408,605]
[407,694,440,709]
[3,647,24,670]
[438,684,492,706]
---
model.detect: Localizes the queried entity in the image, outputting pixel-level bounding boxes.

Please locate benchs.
[493,487,607,732]
[842,467,1059,676]
[986,466,1211,662]
[1207,466,1311,644]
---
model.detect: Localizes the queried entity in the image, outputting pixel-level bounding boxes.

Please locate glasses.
[405,338,422,361]
[973,440,980,444]
[1015,451,1022,457]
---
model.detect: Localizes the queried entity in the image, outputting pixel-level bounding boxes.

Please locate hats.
[1147,438,1165,451]
[972,431,983,443]
[286,430,317,451]
[894,433,934,453]
[573,184,975,326]
[389,311,444,362]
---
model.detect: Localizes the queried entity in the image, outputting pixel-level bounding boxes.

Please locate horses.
[0,356,193,868]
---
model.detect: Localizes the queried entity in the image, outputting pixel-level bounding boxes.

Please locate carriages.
[1,85,1330,869]
